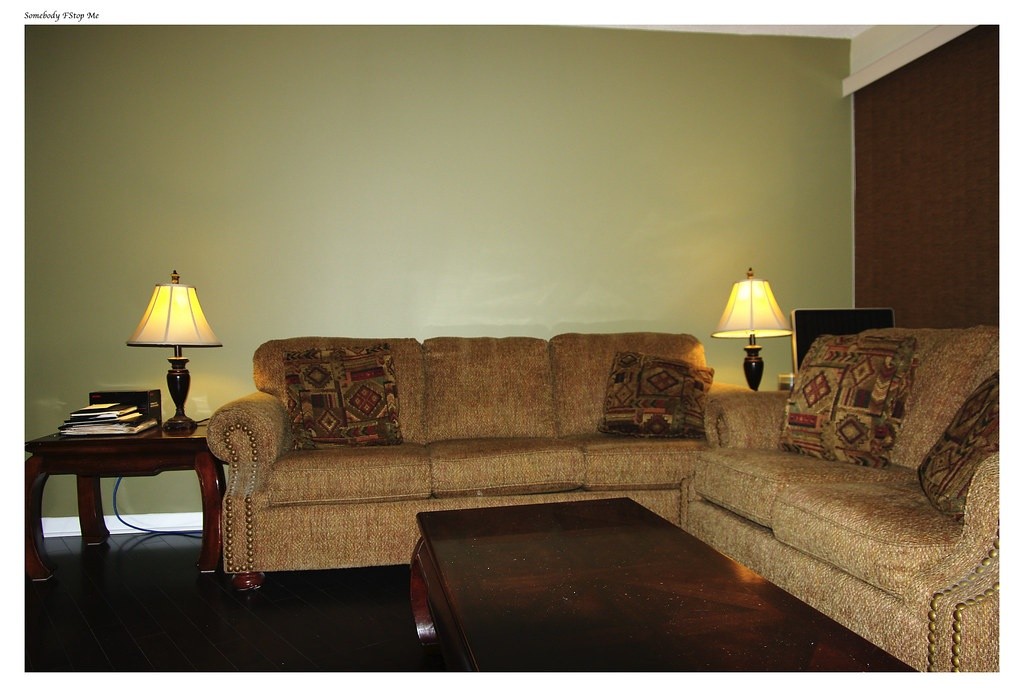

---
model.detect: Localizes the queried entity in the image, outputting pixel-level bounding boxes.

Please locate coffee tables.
[410,496,865,673]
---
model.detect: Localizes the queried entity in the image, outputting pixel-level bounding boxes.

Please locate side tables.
[25,422,225,579]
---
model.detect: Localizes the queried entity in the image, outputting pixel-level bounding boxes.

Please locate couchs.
[207,336,754,587]
[686,327,1001,673]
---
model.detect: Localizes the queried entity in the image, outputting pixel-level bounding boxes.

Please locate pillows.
[281,343,404,449]
[594,351,716,439]
[916,369,999,524]
[776,334,919,465]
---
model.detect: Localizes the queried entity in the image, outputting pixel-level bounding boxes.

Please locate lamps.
[714,267,795,391]
[128,270,224,430]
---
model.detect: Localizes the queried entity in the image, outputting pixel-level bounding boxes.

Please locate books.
[58,403,157,435]
